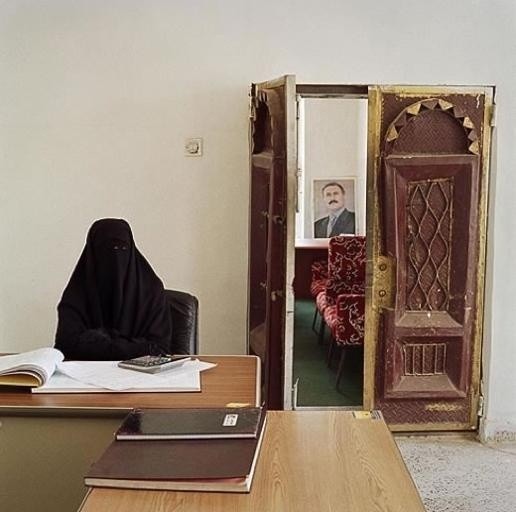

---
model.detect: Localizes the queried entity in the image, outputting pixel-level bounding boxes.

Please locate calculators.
[118,354,191,374]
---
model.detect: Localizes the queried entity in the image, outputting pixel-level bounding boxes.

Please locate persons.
[53,217,174,361]
[314,183,354,238]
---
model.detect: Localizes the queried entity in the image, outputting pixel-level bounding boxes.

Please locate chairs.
[310,236,366,387]
[165,290,198,355]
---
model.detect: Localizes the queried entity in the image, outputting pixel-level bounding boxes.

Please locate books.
[83,408,267,494]
[0,346,202,393]
[115,405,263,440]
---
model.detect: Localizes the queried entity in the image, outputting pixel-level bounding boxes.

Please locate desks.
[0,355,261,512]
[77,410,427,512]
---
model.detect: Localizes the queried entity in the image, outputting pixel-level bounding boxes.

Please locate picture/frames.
[311,177,359,241]
